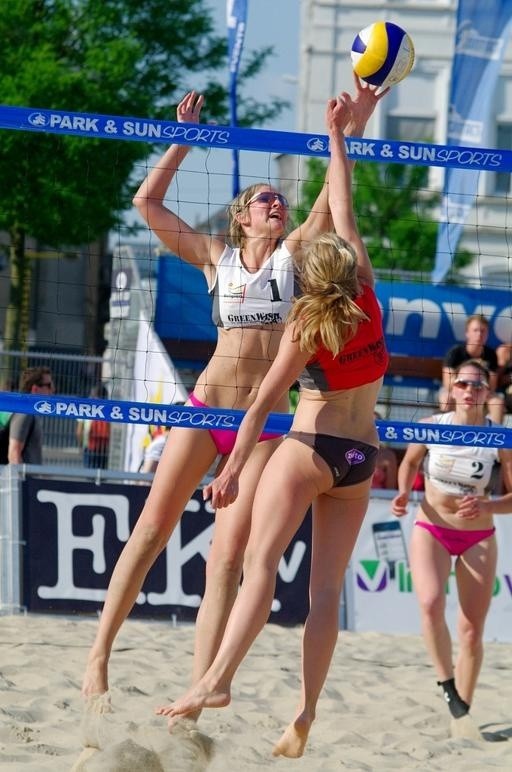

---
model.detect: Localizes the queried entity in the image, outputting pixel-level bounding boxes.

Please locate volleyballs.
[352,18,414,87]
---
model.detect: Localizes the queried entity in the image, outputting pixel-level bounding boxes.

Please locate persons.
[0,367,56,465]
[390,359,512,718]
[76,384,110,470]
[82,69,391,735]
[440,315,512,425]
[156,91,389,759]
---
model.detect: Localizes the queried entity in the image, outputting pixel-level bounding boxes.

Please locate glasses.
[451,380,489,390]
[243,192,289,207]
[40,382,52,389]
